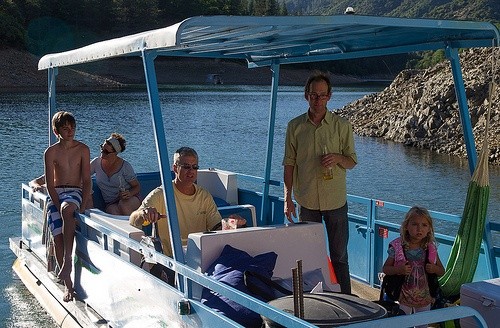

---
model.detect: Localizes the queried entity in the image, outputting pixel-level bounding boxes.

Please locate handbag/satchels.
[399,265,431,307]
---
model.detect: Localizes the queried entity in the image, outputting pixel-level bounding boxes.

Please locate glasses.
[307,92,329,101]
[177,163,200,170]
[100,143,115,154]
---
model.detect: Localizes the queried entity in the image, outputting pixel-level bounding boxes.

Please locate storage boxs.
[459,277,500,327]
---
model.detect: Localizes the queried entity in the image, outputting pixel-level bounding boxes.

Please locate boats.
[7,7,500,328]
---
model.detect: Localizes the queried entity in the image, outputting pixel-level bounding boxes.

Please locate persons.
[383,206,445,328]
[44,111,92,302]
[129,146,223,286]
[28,134,143,216]
[282,75,358,293]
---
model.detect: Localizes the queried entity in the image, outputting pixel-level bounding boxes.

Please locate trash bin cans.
[259,292,387,328]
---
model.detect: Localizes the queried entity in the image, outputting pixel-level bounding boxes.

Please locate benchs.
[92,171,175,220]
[184,220,343,303]
[142,204,257,237]
[331,306,488,328]
